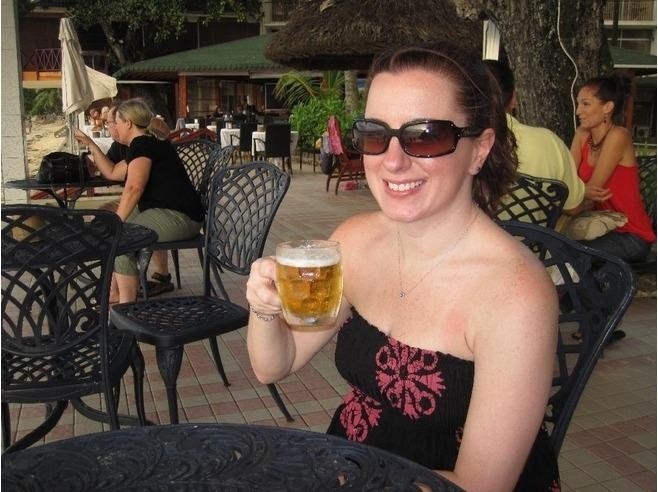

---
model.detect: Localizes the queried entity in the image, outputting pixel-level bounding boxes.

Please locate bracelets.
[249,304,281,322]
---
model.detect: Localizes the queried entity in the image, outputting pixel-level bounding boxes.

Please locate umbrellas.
[58,16,94,155]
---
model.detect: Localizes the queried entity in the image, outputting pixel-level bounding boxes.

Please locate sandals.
[132,273,174,299]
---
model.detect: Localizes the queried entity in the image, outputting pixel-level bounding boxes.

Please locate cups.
[90,119,111,138]
[267,238,344,328]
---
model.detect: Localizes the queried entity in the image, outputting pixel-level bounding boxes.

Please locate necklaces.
[585,123,612,158]
[396,203,481,300]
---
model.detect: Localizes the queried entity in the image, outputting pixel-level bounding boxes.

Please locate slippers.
[572,328,626,348]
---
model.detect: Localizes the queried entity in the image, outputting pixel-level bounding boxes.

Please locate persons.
[209,104,245,122]
[71,96,205,320]
[567,76,656,350]
[246,36,563,492]
[469,59,586,266]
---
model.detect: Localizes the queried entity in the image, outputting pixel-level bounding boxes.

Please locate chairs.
[484,215,638,492]
[177,111,365,196]
[483,170,570,262]
[139,145,235,297]
[111,161,296,427]
[582,156,658,349]
[2,201,147,455]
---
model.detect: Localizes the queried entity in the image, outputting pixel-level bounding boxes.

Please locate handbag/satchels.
[35,151,87,203]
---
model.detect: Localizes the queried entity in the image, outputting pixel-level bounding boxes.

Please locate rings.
[599,200,601,203]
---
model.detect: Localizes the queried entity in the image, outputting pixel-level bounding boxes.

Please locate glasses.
[350,118,485,159]
